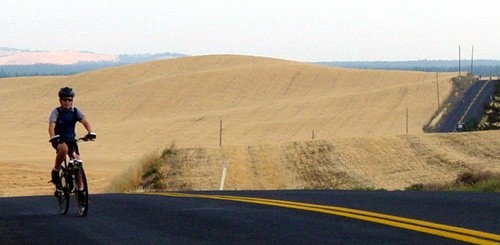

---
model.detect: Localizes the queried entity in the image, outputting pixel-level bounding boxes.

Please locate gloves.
[53,137,61,143]
[87,132,96,140]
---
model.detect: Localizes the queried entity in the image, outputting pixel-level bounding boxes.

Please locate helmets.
[59,87,74,97]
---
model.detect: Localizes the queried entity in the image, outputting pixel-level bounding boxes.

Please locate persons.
[48,87,96,206]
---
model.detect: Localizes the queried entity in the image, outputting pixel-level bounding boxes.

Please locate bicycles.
[48,132,96,217]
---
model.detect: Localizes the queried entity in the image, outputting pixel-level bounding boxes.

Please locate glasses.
[62,98,73,101]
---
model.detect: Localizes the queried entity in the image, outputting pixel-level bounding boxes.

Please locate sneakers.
[52,170,60,184]
[78,190,85,203]
